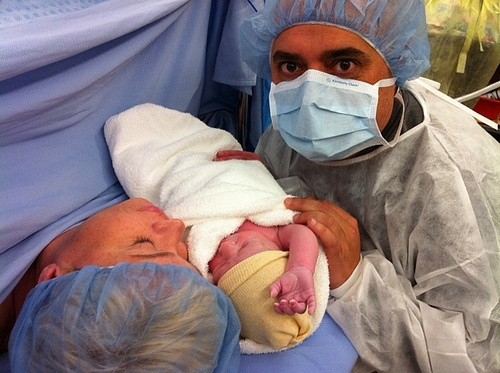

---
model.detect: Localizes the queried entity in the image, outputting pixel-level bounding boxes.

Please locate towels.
[103,102,331,355]
